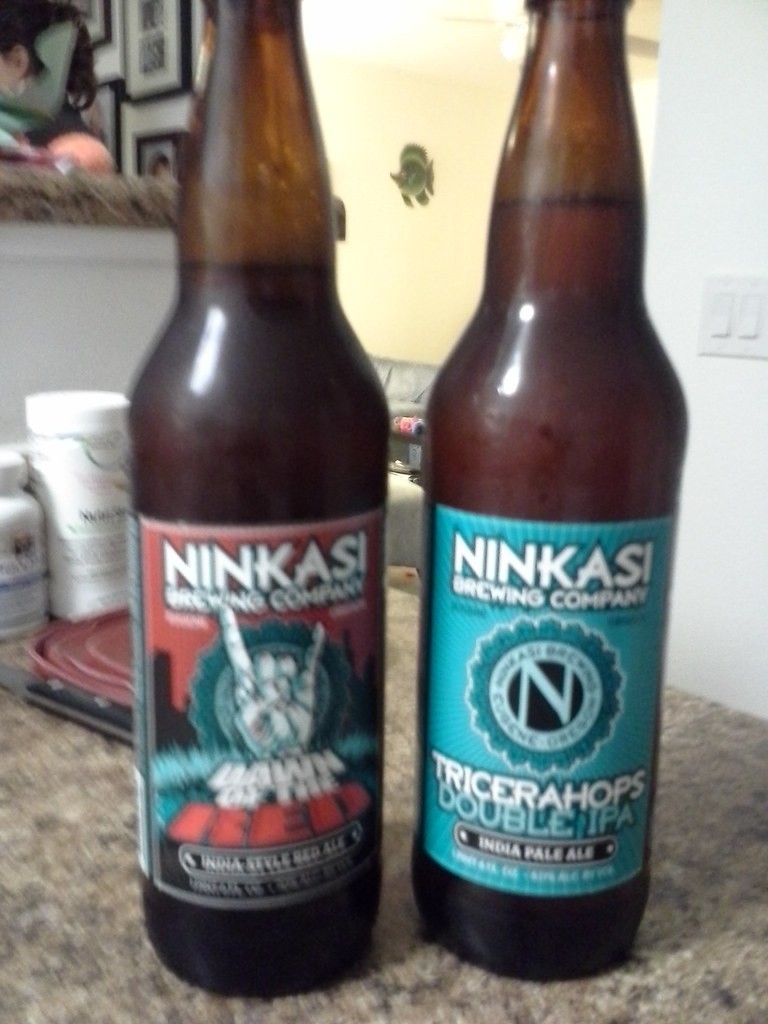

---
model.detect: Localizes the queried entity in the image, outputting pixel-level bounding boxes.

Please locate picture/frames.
[72,0,191,179]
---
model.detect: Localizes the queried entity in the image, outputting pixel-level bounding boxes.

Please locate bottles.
[23,391,131,622]
[0,453,48,645]
[412,0,686,985]
[127,0,392,999]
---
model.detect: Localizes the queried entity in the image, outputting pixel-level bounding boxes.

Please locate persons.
[0,0,113,177]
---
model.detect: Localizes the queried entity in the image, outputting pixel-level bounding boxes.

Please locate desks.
[0,589,768,1024]
